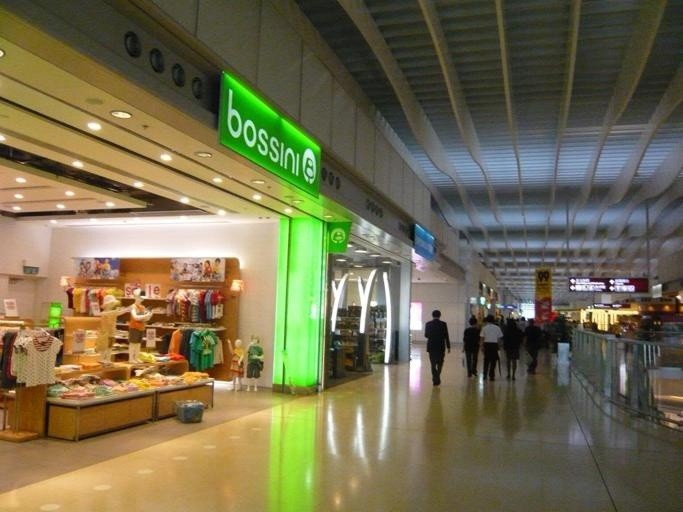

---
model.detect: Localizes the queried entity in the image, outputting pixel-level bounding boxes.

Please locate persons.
[128,288,153,364]
[170,259,222,282]
[227,339,245,391]
[79,258,110,280]
[99,295,133,364]
[503,319,525,379]
[461,318,480,377]
[245,334,264,392]
[425,310,451,385]
[479,316,504,381]
[523,319,542,374]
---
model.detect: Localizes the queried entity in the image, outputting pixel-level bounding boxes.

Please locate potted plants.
[549,315,575,361]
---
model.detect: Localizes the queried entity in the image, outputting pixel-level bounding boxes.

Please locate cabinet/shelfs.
[67,255,238,384]
[326,304,388,380]
[0,316,218,444]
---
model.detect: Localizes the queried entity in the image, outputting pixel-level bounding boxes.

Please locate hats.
[134,289,148,299]
[101,295,122,308]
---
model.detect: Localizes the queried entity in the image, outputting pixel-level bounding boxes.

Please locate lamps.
[228,278,247,299]
[57,274,74,292]
